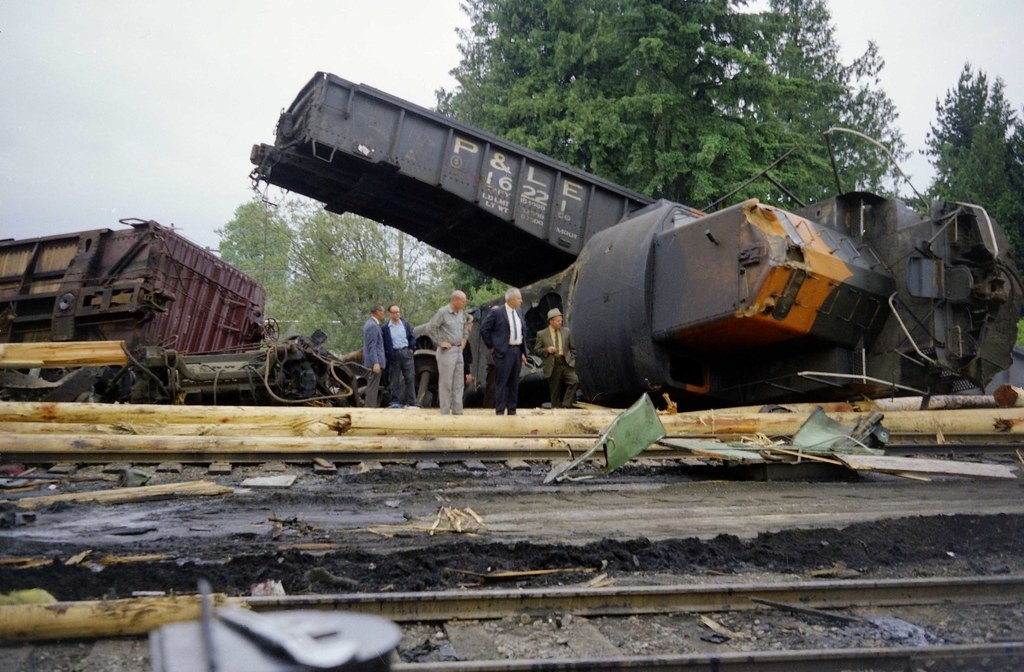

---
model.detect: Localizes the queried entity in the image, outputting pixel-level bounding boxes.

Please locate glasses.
[390,311,399,315]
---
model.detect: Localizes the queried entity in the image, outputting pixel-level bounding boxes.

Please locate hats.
[547,308,563,321]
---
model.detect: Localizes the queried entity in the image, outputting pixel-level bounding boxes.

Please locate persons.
[381,304,422,408]
[535,308,579,409]
[463,314,475,407]
[480,287,529,415]
[425,290,469,415]
[363,304,386,408]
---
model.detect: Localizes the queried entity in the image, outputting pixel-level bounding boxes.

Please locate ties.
[554,329,559,359]
[512,310,517,340]
